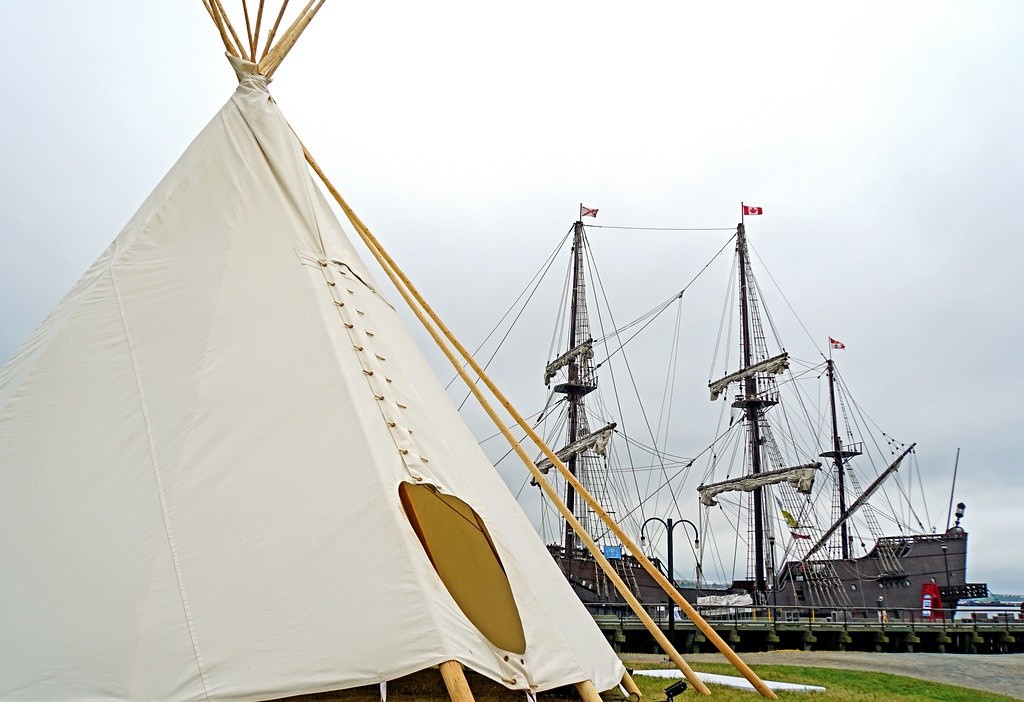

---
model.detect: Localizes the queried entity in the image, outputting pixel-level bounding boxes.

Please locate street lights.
[640,518,699,660]
[768,535,777,626]
[941,541,954,624]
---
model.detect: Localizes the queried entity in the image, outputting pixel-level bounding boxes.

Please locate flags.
[580,206,599,218]
[782,510,796,527]
[829,338,845,349]
[742,206,763,215]
[775,496,783,510]
[790,531,811,541]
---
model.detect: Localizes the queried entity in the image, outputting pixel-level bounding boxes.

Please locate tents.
[0,0,781,702]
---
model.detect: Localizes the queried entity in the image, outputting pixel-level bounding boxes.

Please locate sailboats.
[441,201,968,622]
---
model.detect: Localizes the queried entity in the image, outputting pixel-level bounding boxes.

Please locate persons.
[546,542,559,554]
[877,596,886,623]
[583,546,604,560]
